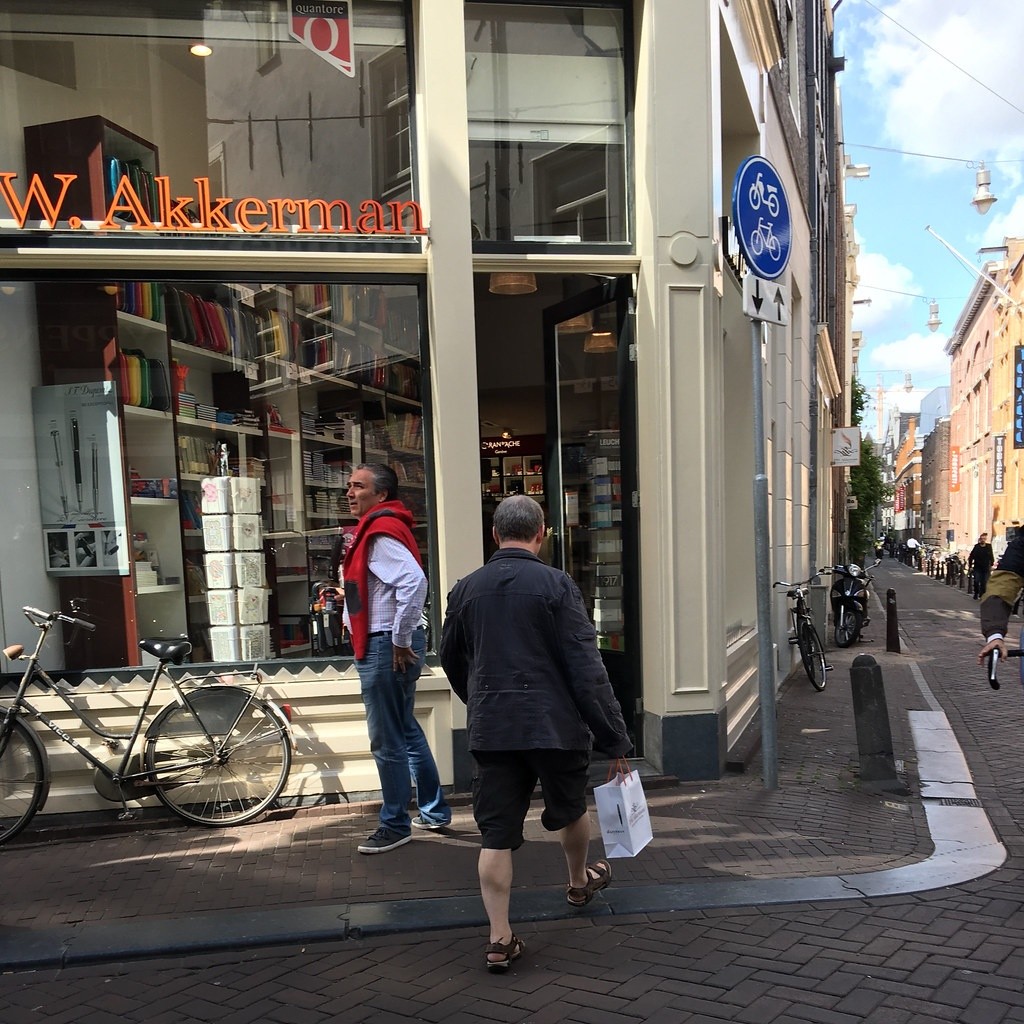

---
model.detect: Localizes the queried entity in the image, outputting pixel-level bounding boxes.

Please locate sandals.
[566,857,611,906]
[485,931,524,973]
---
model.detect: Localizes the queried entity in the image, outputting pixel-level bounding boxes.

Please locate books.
[173,392,424,529]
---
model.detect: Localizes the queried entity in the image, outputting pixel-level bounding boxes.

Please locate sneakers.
[358,827,412,853]
[410,816,444,830]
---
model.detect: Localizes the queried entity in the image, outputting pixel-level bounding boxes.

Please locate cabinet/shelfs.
[23,114,431,672]
[480,431,589,565]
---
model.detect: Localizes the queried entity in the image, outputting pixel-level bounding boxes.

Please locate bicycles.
[773,568,834,692]
[0,604,299,845]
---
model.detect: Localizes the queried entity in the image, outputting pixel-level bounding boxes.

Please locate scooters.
[874,544,885,560]
[823,558,881,649]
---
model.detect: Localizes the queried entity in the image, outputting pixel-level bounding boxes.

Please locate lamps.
[902,373,913,393]
[558,311,594,334]
[583,310,618,353]
[489,272,537,295]
[970,161,998,215]
[925,297,942,331]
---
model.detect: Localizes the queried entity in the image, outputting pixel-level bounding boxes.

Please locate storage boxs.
[581,431,624,632]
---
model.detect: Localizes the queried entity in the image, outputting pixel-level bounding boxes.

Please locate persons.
[967,533,1024,621]
[439,495,634,973]
[318,462,451,854]
[874,535,925,567]
[978,524,1024,668]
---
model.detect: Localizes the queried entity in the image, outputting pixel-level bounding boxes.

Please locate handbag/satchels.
[594,755,653,858]
